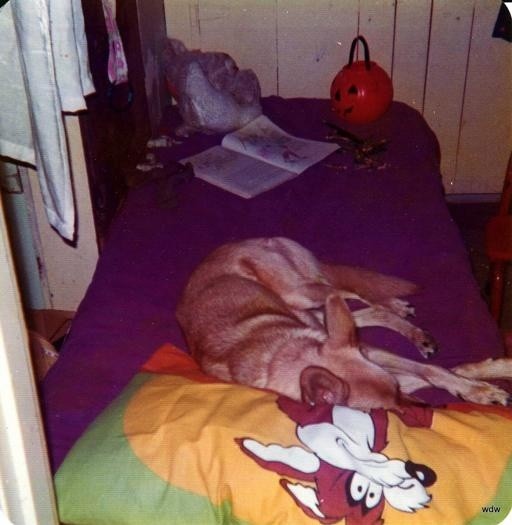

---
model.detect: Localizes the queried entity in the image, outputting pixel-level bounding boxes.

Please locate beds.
[40,96,512,523]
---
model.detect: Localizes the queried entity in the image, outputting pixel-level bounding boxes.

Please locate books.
[177,113,341,202]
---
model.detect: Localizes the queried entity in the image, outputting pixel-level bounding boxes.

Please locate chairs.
[484,156,512,323]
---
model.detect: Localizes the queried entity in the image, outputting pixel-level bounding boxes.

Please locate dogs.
[173,238,512,412]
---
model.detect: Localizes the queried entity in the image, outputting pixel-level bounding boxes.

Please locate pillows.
[52,344,512,525]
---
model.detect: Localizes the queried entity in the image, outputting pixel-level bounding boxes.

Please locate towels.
[0,0,95,246]
